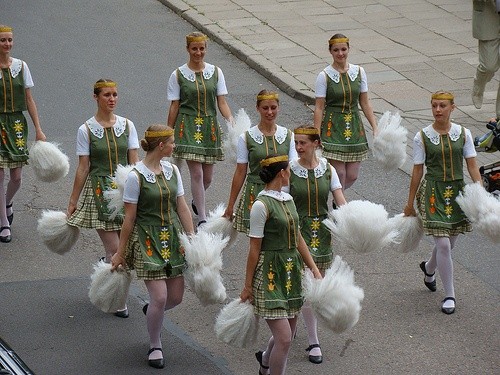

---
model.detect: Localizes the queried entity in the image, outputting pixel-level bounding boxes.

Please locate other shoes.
[255,351,270,375]
[332,199,336,209]
[0,226,11,242]
[99,257,105,262]
[305,344,323,364]
[114,310,129,318]
[496,107,500,119]
[147,347,164,369]
[192,200,199,215]
[420,260,437,292]
[472,82,483,109]
[6,201,13,225]
[142,303,161,338]
[198,220,206,226]
[441,296,456,314]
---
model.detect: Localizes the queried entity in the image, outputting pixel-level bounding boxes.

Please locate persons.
[288,126,348,363]
[110,124,194,368]
[223,90,295,237]
[314,34,377,211]
[239,155,322,375]
[472,0,500,120]
[0,24,46,242]
[167,32,231,226]
[404,91,483,315]
[68,79,139,319]
